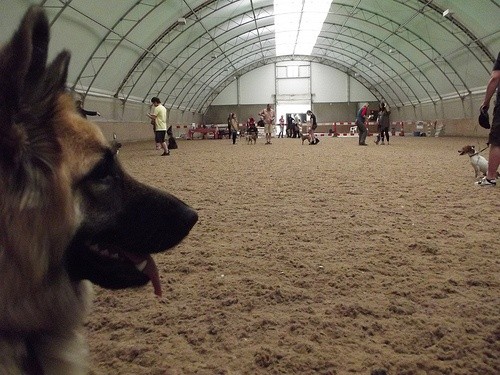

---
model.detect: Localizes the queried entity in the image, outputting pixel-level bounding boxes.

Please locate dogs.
[458,144,500,179]
[246,134,257,145]
[1,3,199,374]
[299,132,311,145]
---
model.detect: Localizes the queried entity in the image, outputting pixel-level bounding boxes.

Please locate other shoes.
[374,141,378,145]
[315,139,319,144]
[360,143,368,145]
[380,143,385,145]
[388,143,389,145]
[309,141,315,144]
[161,150,170,156]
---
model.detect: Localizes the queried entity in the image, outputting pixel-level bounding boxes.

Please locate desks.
[188,129,220,140]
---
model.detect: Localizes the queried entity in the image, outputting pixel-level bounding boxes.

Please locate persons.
[247,117,258,138]
[355,103,370,146]
[278,116,284,138]
[286,114,302,138]
[75,100,101,120]
[307,110,320,145]
[376,102,390,145]
[145,97,171,156]
[474,50,500,187]
[258,104,276,144]
[228,113,238,144]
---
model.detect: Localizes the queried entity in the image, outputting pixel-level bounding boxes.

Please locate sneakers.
[474,176,496,186]
[496,172,500,178]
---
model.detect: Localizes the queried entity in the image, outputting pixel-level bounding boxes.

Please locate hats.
[478,107,490,129]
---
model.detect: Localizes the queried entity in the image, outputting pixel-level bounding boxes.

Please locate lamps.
[442,9,454,17]
[389,49,396,53]
[178,18,186,25]
